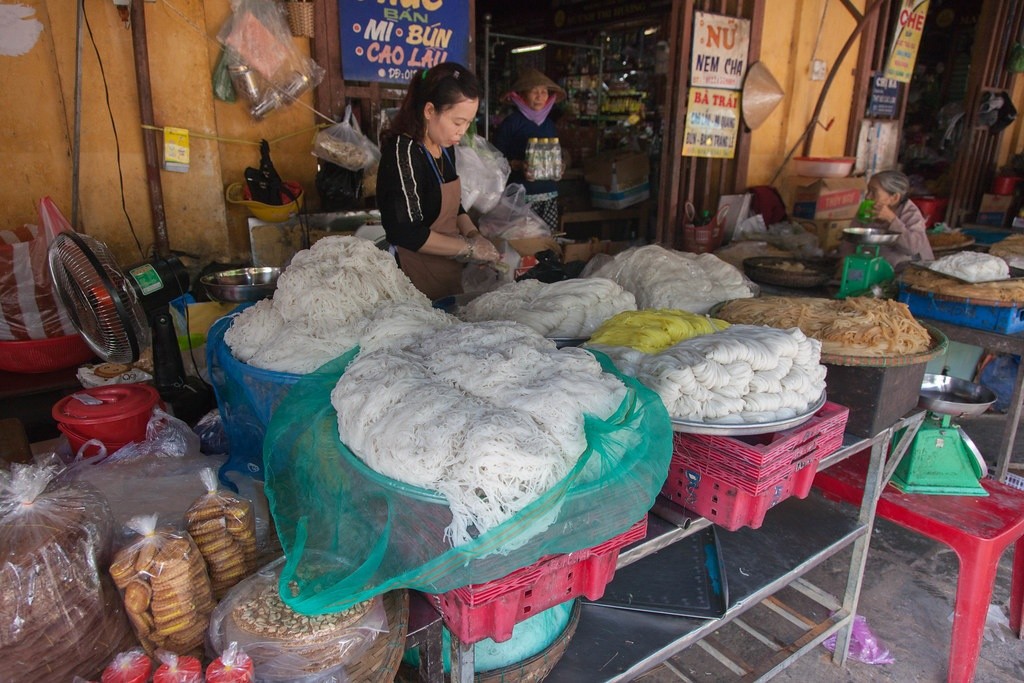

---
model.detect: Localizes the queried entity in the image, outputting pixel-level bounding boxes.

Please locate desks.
[908,312,1024,485]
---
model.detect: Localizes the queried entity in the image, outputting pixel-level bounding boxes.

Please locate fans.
[47,228,218,426]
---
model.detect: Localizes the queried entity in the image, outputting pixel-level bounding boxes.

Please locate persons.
[491,68,568,241]
[376,61,501,302]
[840,170,936,271]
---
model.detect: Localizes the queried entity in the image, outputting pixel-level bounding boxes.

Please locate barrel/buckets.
[53,384,166,465]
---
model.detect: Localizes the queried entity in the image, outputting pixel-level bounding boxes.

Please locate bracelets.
[462,234,473,262]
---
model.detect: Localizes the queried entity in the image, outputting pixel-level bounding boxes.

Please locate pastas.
[223,234,1024,564]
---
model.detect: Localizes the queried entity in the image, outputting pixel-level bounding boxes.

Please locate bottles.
[525,137,563,182]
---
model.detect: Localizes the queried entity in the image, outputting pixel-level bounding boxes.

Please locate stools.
[808,448,1024,683]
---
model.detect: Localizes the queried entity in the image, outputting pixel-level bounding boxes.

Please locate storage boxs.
[374,490,652,652]
[659,390,854,538]
[902,269,1022,339]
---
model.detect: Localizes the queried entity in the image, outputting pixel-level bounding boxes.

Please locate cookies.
[111,496,257,663]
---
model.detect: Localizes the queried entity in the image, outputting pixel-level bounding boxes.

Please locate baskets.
[241,291,850,683]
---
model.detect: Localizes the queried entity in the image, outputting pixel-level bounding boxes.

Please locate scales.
[888,374,998,496]
[833,227,902,301]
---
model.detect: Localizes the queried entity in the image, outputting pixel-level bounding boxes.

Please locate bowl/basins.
[200,265,284,309]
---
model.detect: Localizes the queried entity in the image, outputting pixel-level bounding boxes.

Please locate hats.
[502,66,567,106]
